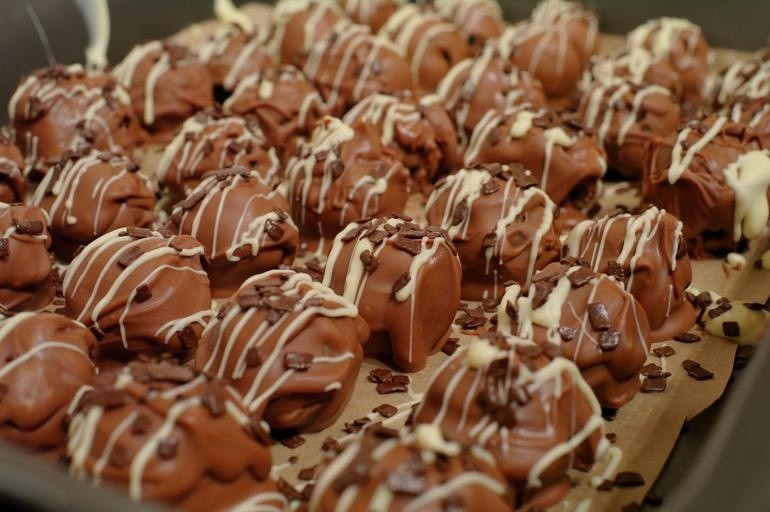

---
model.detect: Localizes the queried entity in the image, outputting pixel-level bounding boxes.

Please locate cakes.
[0,0,770,512]
